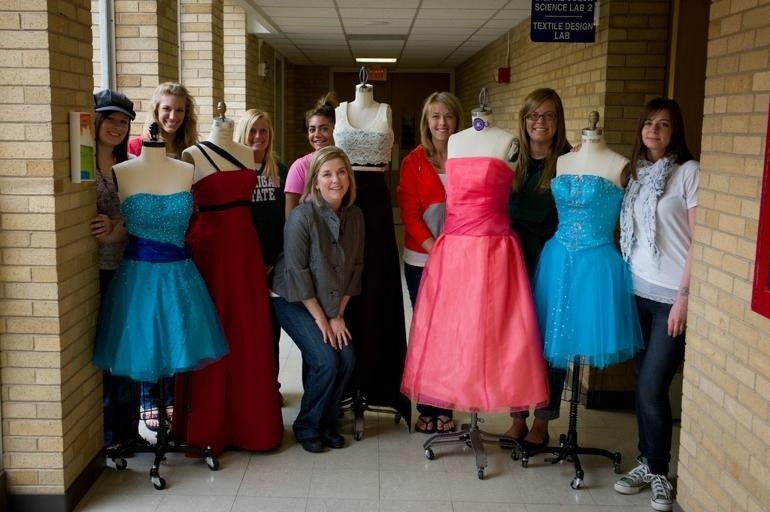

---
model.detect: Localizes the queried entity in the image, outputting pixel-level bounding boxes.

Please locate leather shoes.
[298,429,346,451]
[499,426,550,459]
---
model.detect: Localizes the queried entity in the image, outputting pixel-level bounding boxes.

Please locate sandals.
[415,412,458,434]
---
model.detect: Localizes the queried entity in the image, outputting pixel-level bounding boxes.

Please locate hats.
[92,89,136,121]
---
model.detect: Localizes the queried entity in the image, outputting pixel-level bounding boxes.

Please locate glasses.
[524,110,559,121]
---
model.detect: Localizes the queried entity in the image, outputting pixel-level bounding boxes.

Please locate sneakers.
[613,457,674,510]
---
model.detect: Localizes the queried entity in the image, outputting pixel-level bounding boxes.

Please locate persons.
[180,117,285,461]
[612,97,705,511]
[237,92,365,453]
[332,86,408,405]
[401,107,549,413]
[93,88,149,459]
[534,129,646,369]
[92,138,228,386]
[499,90,572,461]
[139,80,201,433]
[395,93,465,433]
